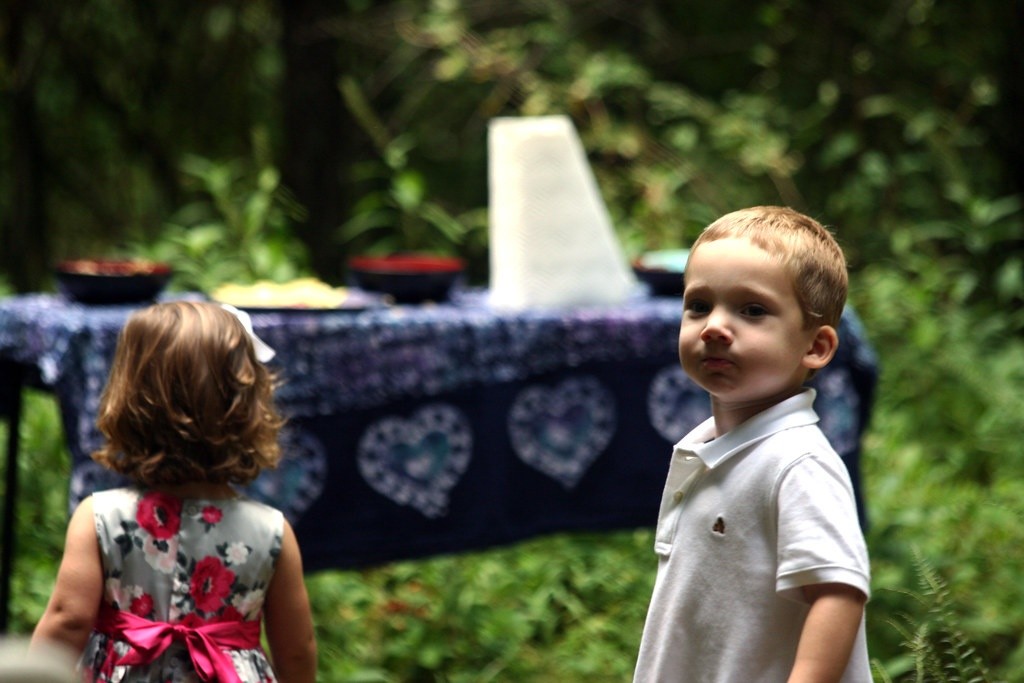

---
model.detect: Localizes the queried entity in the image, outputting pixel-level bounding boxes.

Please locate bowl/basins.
[53,257,178,306]
[632,245,695,291]
[351,251,459,303]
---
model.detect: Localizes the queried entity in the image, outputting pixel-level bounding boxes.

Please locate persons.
[631,206,876,683]
[26,296,321,683]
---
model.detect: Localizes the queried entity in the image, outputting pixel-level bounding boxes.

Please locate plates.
[209,273,379,311]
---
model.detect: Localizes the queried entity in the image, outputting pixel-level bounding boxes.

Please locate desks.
[0,288,880,638]
